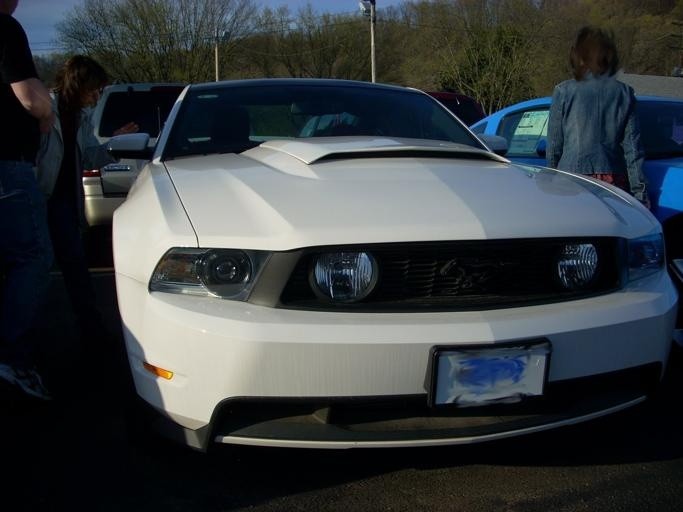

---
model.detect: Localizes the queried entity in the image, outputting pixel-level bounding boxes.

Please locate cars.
[296,88,488,139]
[469,93,683,221]
[79,84,187,227]
[113,72,683,462]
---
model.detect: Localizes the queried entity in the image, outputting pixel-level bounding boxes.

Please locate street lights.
[213,27,229,82]
[359,1,377,86]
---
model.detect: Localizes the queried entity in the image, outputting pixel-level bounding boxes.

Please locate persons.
[35,55,139,347]
[0,0,56,402]
[545,24,652,210]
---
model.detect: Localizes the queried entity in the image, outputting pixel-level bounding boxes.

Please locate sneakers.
[0,362,52,401]
